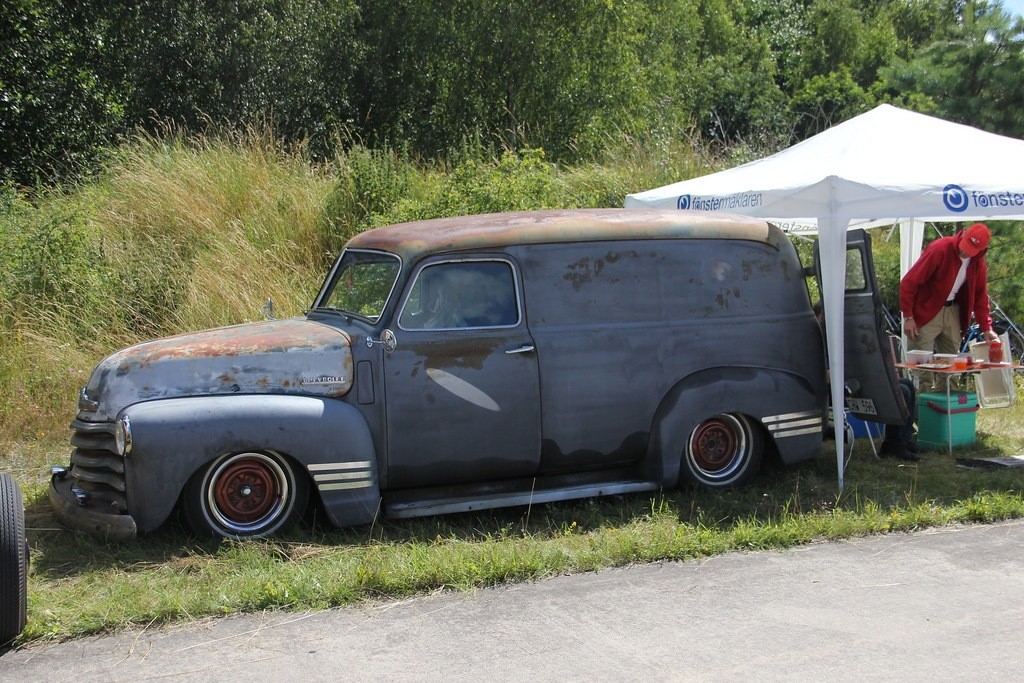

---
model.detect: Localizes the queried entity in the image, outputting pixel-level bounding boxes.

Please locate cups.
[955,357,967,369]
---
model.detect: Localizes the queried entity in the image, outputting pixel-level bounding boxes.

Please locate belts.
[943,300,954,308]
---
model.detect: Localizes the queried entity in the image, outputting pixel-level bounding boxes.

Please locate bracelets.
[904,316,913,320]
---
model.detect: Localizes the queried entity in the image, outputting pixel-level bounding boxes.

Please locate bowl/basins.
[905,350,958,365]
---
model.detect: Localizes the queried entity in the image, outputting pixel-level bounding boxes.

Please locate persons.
[813,298,920,462]
[899,223,1002,425]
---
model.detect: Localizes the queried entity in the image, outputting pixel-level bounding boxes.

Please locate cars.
[46,208,909,551]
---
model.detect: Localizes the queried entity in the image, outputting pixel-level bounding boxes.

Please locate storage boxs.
[913,391,980,452]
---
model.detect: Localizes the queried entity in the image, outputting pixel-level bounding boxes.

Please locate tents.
[624,102,1023,499]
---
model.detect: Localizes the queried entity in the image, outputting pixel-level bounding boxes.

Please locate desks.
[894,360,1024,456]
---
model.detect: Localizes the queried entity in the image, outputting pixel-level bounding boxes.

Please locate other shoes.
[882,441,929,460]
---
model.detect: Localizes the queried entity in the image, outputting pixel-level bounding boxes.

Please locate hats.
[959,223,990,255]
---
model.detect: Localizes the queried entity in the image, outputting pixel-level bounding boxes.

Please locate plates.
[915,362,952,369]
